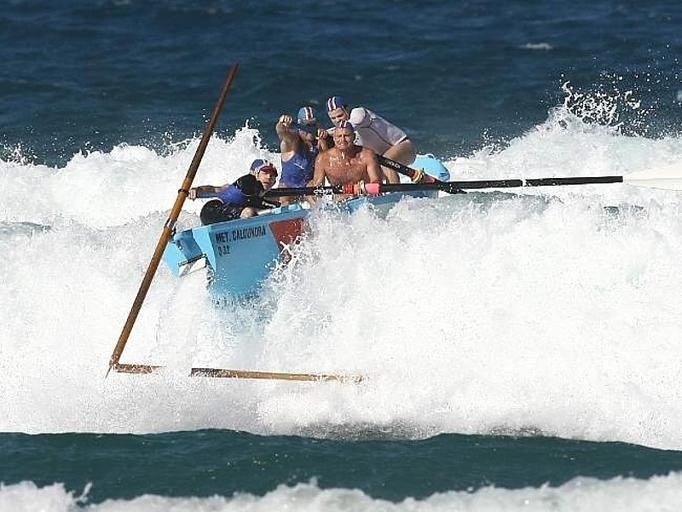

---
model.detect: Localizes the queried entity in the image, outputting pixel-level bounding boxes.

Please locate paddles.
[187,162,682,199]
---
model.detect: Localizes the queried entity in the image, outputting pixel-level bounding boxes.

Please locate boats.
[161,154,450,312]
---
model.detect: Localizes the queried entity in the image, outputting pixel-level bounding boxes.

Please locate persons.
[306,120,382,188]
[324,96,412,184]
[297,107,326,131]
[275,114,317,206]
[188,159,277,219]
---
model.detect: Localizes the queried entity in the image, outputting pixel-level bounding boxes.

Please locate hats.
[334,122,355,130]
[255,163,278,180]
[324,96,348,115]
[296,106,320,122]
[250,158,268,172]
